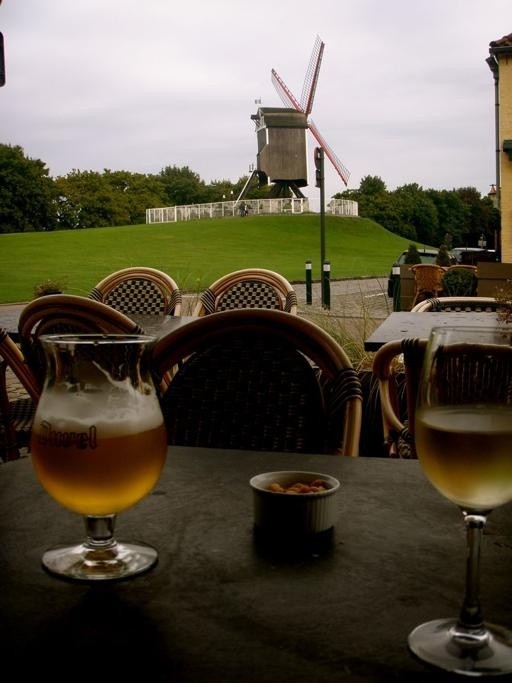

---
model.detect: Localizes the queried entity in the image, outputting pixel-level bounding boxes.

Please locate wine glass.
[407,324,512,677]
[27,333,168,580]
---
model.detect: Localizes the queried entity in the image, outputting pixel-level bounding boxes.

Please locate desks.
[365,309,510,352]
[2,312,201,341]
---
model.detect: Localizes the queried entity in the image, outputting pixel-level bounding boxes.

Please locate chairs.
[142,307,365,458]
[410,296,510,315]
[372,337,510,458]
[0,292,149,461]
[87,266,183,317]
[192,267,298,317]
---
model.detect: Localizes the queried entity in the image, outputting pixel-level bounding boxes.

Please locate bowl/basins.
[248,470,341,535]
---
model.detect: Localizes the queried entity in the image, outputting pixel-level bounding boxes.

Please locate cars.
[388,248,495,297]
